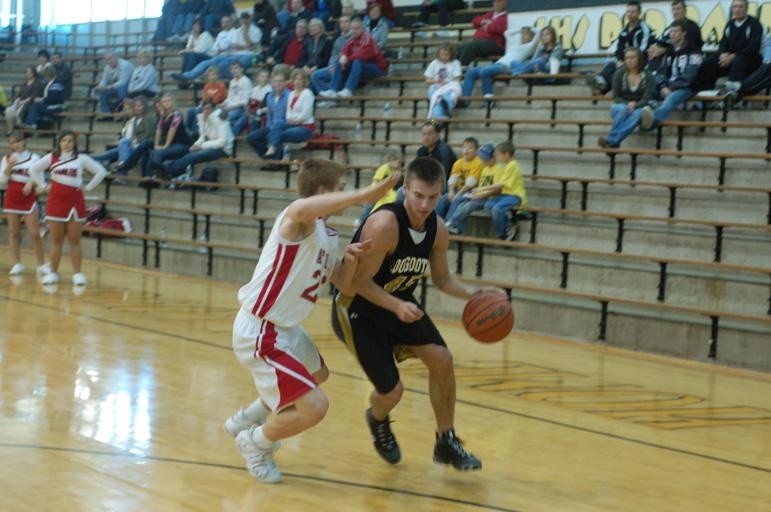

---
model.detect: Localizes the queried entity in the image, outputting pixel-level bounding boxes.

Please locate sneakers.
[71,271,87,286]
[504,222,520,242]
[222,404,282,486]
[314,87,354,110]
[362,406,405,466]
[8,261,25,277]
[442,219,464,236]
[34,261,61,288]
[431,426,487,473]
[636,106,656,132]
[595,135,620,155]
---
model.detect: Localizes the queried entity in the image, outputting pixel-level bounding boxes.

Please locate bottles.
[282,143,291,162]
[176,174,187,180]
[159,224,168,248]
[355,119,362,139]
[184,163,193,183]
[199,230,209,253]
[384,100,391,118]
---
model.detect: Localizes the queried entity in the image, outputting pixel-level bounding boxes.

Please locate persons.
[0,129,51,275]
[27,128,107,286]
[331,157,484,471]
[223,159,402,483]
[2,0,771,242]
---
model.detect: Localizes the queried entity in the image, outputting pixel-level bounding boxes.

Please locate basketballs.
[463,293,515,343]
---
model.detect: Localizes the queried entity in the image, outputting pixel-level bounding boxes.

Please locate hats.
[478,141,494,161]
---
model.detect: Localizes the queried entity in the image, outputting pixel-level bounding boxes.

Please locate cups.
[549,46,561,74]
